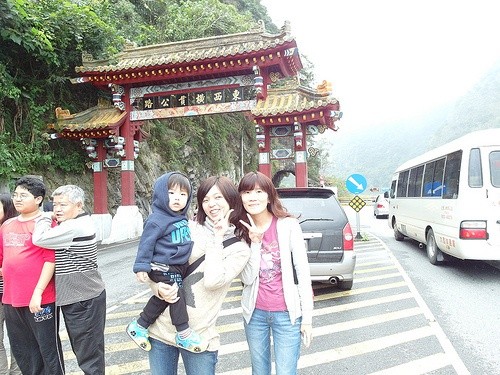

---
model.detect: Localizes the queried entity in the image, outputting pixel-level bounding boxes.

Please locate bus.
[383,129,500,268]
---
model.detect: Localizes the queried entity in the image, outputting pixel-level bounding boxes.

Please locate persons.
[125,171,211,354]
[0,194,23,375]
[32,185,106,375]
[228,170,314,375]
[0,175,65,375]
[148,175,251,375]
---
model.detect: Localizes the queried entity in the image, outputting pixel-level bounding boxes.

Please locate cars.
[373,194,390,219]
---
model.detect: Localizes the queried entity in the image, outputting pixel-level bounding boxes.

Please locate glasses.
[11,194,36,199]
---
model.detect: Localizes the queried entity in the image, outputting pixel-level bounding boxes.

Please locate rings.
[165,297,169,300]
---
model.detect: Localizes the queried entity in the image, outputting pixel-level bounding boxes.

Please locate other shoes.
[175,329,210,353]
[126,317,152,352]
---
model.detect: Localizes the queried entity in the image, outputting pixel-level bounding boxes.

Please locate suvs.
[272,185,357,291]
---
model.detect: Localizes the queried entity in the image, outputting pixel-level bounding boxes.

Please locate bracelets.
[35,287,45,291]
